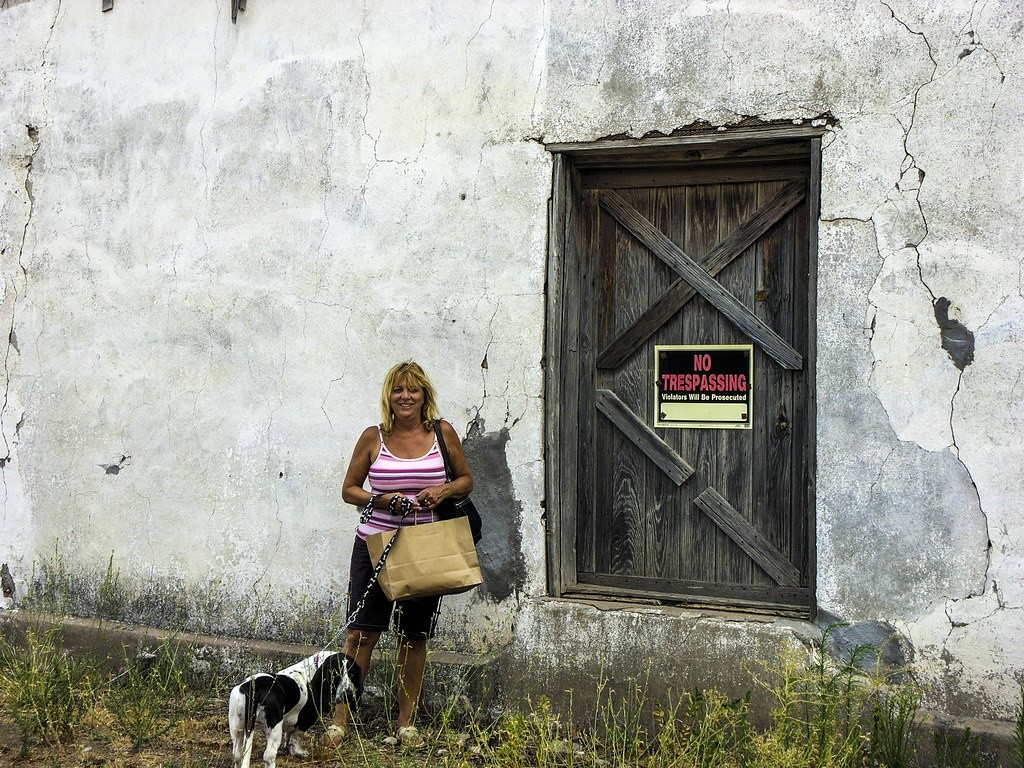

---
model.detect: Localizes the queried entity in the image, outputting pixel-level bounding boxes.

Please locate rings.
[424,499,431,505]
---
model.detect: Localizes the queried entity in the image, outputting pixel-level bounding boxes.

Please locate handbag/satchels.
[365,500,483,603]
[437,477,482,546]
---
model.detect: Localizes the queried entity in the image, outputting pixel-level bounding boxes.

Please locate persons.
[321,361,474,750]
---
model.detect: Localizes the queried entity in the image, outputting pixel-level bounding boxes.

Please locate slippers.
[395,726,424,748]
[322,725,347,749]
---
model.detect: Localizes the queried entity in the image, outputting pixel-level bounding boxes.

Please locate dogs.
[228,649,365,768]
